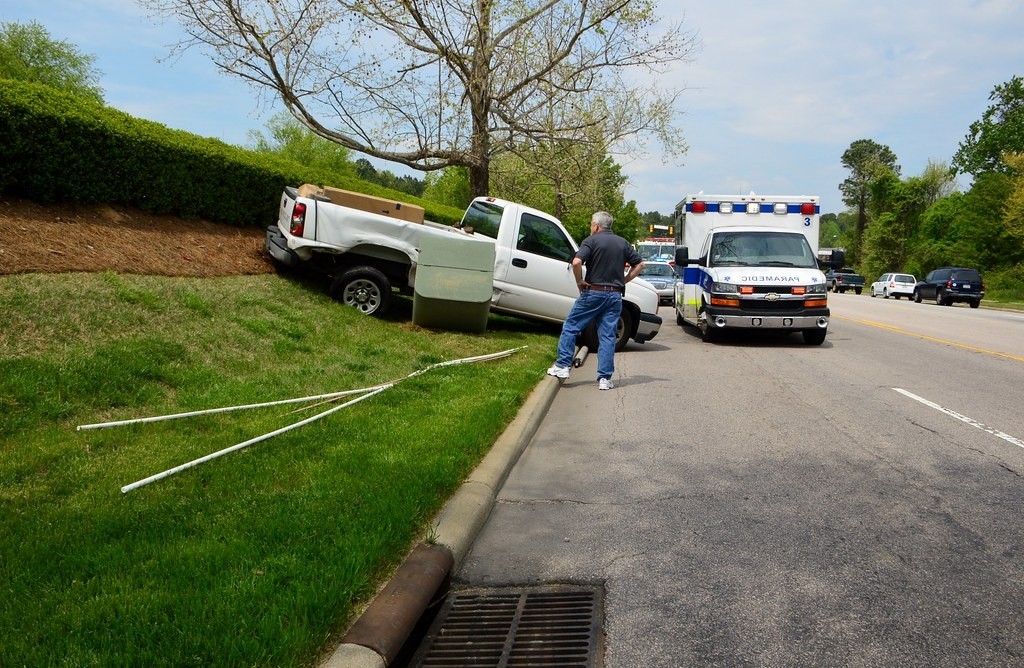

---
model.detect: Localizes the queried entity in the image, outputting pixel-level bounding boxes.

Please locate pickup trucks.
[265,185,663,352]
[823,267,867,296]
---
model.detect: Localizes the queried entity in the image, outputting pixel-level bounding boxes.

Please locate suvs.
[913,266,986,308]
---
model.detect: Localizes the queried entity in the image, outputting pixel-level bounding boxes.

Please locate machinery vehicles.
[628,223,676,273]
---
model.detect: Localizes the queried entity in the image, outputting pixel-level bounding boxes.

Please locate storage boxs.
[322,185,426,225]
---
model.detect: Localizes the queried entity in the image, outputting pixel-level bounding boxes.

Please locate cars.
[870,272,918,301]
[627,260,677,305]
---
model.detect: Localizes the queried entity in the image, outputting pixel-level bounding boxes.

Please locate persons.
[546,211,645,390]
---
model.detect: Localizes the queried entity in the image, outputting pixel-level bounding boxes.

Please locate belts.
[582,286,619,291]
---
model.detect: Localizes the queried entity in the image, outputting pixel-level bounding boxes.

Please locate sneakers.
[547,364,570,379]
[598,377,614,390]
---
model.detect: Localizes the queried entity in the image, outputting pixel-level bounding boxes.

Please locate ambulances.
[671,190,845,347]
